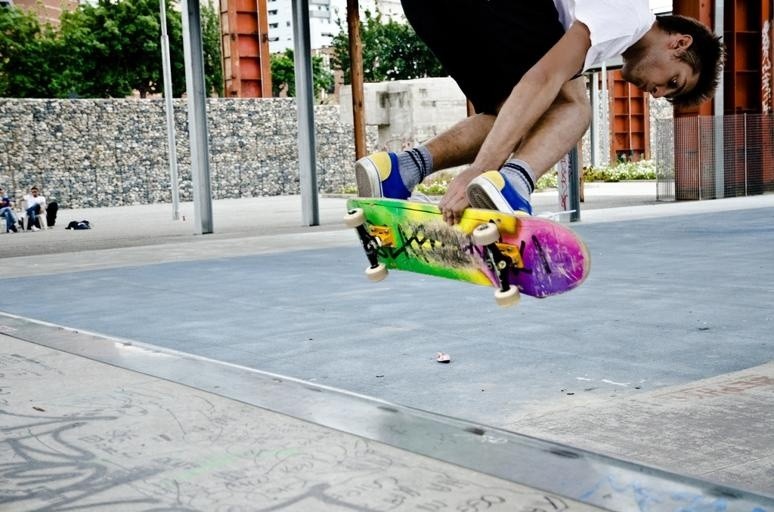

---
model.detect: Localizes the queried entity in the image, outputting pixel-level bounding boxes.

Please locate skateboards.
[346,196,591,307]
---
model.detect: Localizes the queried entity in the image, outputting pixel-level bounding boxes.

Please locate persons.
[353,0,726,214]
[13,186,48,232]
[0,188,18,232]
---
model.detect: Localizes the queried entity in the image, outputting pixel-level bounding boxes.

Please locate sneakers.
[20,211,28,219]
[7,224,18,232]
[354,152,411,200]
[31,225,40,232]
[464,170,532,216]
